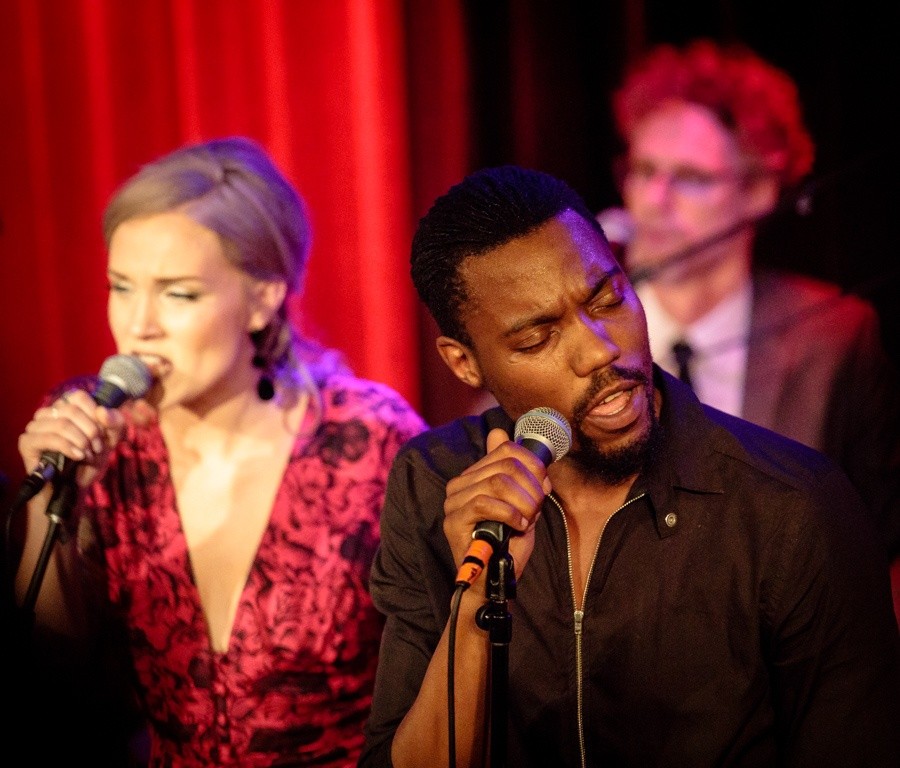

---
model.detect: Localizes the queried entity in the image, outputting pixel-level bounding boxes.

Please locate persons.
[17,44,900,768]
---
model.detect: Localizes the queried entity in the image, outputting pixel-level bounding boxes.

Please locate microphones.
[596,206,636,263]
[11,354,152,511]
[453,405,574,588]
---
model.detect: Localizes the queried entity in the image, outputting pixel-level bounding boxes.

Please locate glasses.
[620,159,758,203]
